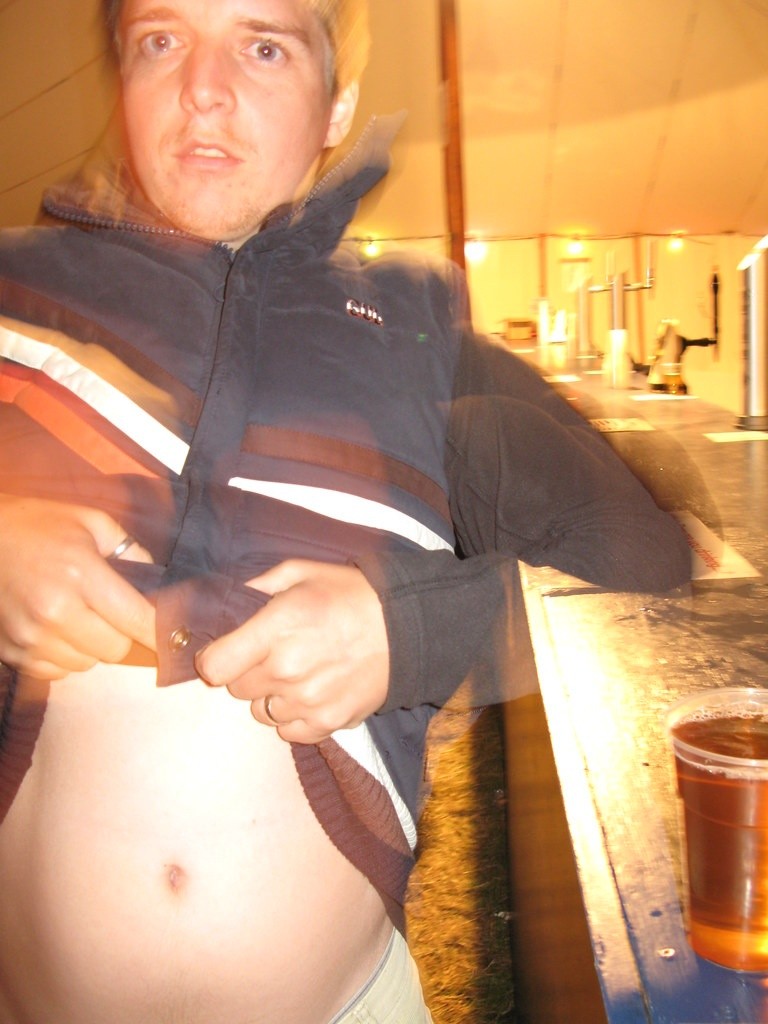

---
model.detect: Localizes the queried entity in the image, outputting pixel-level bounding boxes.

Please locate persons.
[0,0,691,1024]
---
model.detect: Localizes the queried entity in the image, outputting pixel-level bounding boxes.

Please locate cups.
[662,687,768,974]
[659,363,682,393]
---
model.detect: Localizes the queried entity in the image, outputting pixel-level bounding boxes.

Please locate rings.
[264,694,285,724]
[105,535,137,559]
